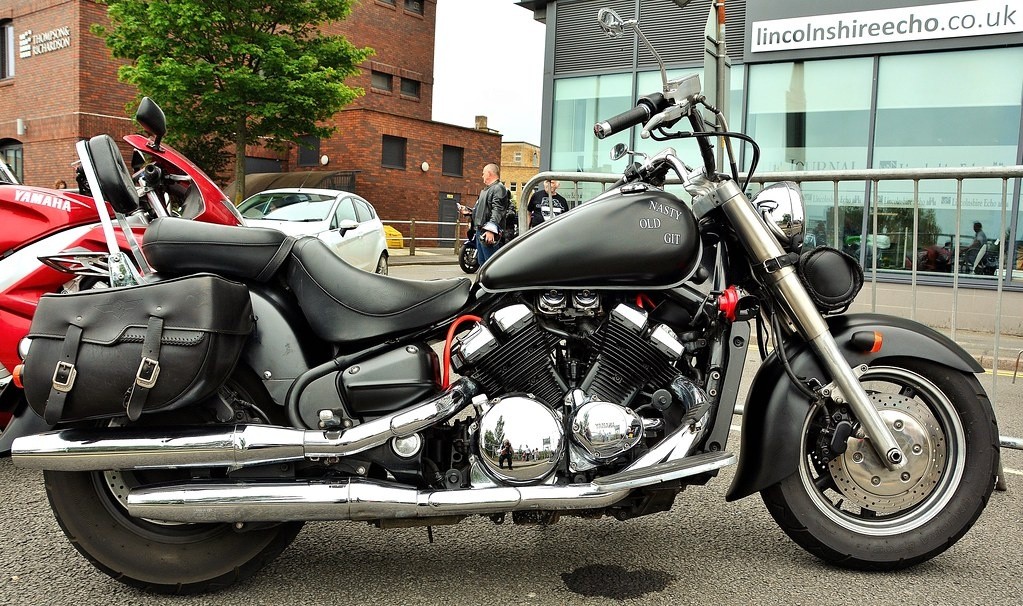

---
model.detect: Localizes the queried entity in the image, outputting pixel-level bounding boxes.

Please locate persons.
[527,179,570,228]
[968,220,988,253]
[458,164,508,267]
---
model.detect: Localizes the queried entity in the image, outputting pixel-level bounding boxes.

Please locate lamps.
[320,154,329,166]
[17,118,27,136]
[421,161,430,172]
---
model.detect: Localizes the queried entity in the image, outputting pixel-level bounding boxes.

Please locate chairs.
[965,242,999,276]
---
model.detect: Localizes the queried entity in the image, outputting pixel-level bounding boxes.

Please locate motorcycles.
[0,97,248,399]
[455,203,534,274]
[0,7,1003,593]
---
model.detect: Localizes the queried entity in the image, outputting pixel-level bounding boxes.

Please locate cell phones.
[456,203,461,209]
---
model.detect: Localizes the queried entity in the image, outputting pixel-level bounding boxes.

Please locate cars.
[234,188,389,276]
